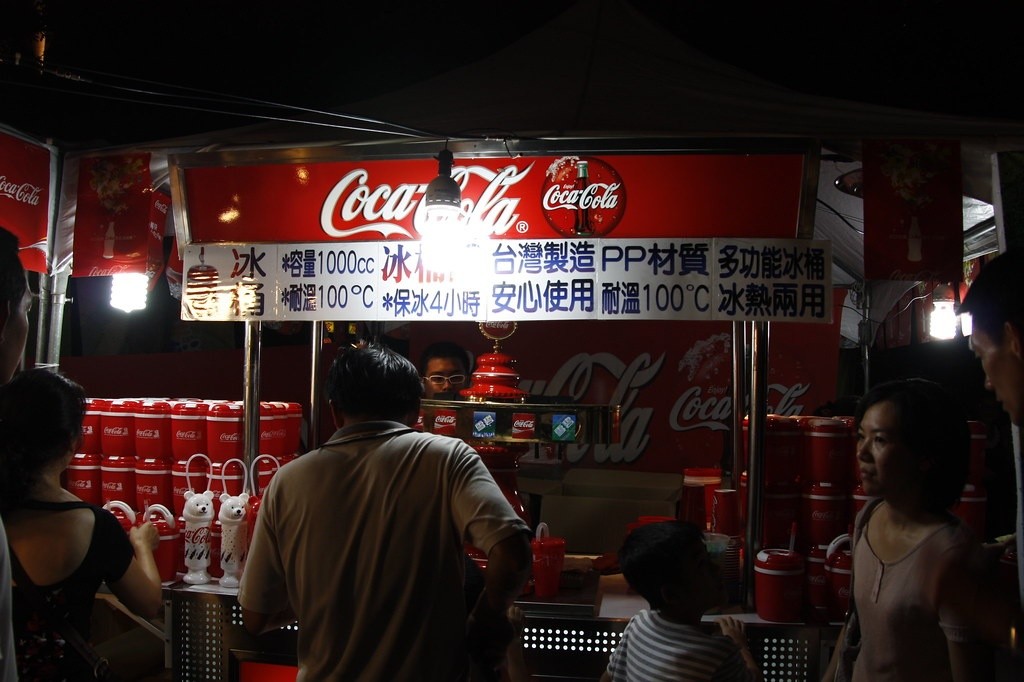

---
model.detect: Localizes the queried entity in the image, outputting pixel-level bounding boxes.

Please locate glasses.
[422,374,467,384]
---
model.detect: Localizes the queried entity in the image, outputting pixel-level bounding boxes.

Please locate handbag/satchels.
[834,498,883,682]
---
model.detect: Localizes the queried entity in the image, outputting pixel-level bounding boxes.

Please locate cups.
[530,523,566,601]
[66,396,303,583]
[684,413,986,623]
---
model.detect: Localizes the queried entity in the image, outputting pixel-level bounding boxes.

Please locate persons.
[958,247,1024,426]
[0,227,162,682]
[237,344,532,682]
[418,343,471,401]
[600,520,760,682]
[819,380,997,682]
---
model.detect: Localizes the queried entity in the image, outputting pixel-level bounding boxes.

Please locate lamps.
[420,151,465,273]
[107,273,149,314]
[928,283,957,339]
